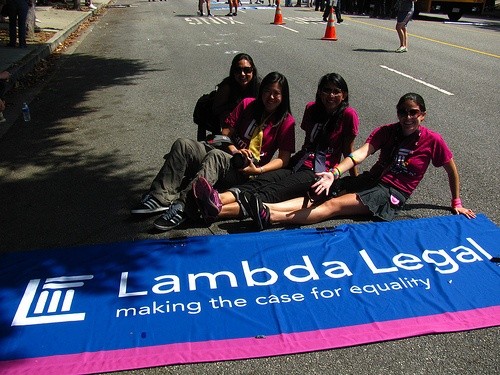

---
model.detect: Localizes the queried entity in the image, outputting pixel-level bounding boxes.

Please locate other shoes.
[233,13,237,16]
[208,14,212,16]
[225,13,232,16]
[200,13,203,16]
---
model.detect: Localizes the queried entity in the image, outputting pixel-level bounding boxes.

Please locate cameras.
[231,153,254,169]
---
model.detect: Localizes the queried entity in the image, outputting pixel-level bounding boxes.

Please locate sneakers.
[395,47,407,53]
[153,203,187,230]
[192,177,223,224]
[131,193,169,214]
[239,190,272,230]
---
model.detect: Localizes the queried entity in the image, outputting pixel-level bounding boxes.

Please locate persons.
[211,54,264,122]
[132,71,296,230]
[199,0,212,16]
[192,73,360,226]
[238,93,477,231]
[225,0,237,16]
[314,0,344,23]
[5,0,33,48]
[396,0,416,53]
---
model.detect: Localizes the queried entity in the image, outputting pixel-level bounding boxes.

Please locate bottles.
[21,102,31,121]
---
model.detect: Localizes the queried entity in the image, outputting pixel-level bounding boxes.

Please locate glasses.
[234,67,252,73]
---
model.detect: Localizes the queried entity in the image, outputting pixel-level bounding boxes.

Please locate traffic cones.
[270,0,287,25]
[322,6,338,40]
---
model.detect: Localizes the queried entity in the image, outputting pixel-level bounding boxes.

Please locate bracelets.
[333,164,343,176]
[260,167,263,174]
[450,198,462,208]
[329,169,338,180]
[347,154,358,166]
